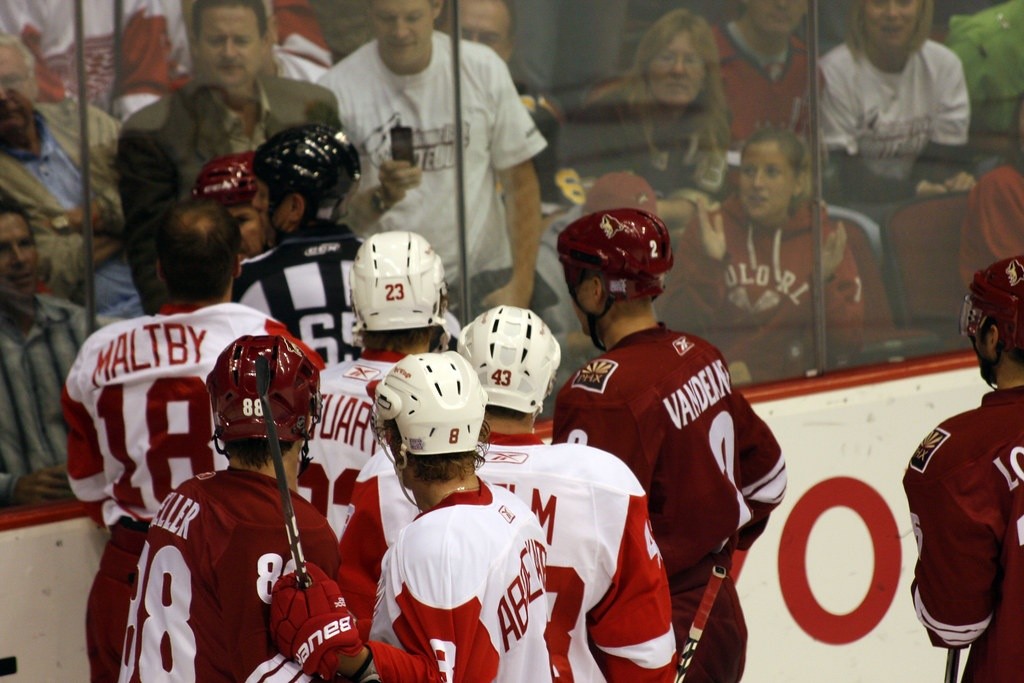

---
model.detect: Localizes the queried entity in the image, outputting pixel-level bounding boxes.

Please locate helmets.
[191,150,257,207]
[349,230,448,331]
[370,351,488,470]
[253,122,361,235]
[457,304,561,418]
[558,207,674,320]
[206,335,323,444]
[958,254,1024,380]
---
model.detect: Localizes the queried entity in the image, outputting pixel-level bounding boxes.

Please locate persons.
[0,1,1024,683]
[549,208,788,683]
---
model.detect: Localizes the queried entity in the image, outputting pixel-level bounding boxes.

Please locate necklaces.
[441,486,479,503]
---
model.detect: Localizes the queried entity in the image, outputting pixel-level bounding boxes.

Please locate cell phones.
[391,127,415,167]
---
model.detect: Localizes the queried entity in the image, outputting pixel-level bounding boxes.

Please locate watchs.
[369,185,390,212]
[50,210,71,234]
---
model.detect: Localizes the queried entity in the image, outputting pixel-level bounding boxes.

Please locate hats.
[583,170,657,216]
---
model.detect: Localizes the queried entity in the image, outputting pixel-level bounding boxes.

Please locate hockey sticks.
[944,648,960,683]
[674,566,727,683]
[255,356,309,587]
